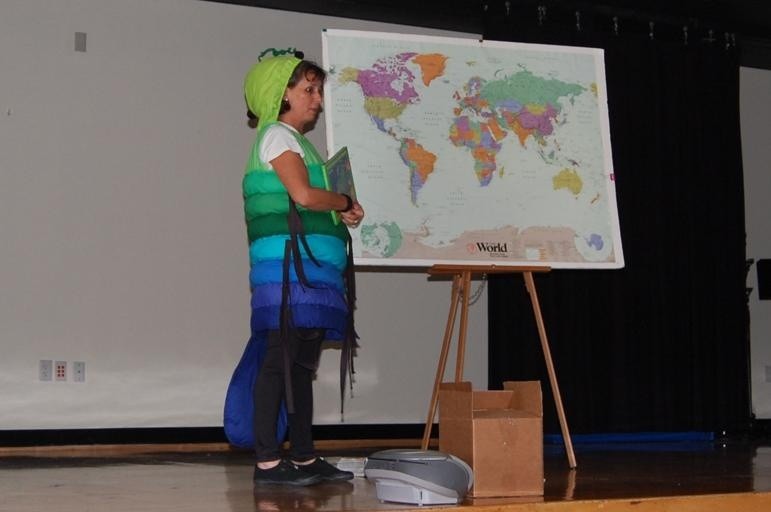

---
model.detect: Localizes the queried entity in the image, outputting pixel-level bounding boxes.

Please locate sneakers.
[250,459,327,488]
[285,456,356,487]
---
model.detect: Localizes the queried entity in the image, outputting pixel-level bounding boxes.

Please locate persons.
[245,53,368,487]
[250,482,357,511]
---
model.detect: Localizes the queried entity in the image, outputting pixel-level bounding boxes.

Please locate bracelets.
[337,194,355,213]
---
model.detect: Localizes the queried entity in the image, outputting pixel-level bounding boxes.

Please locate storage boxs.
[434,381,543,498]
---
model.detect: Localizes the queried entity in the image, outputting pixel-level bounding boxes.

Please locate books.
[322,145,360,228]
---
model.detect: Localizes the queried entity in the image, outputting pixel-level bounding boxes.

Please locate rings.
[352,224,357,228]
[354,219,359,226]
